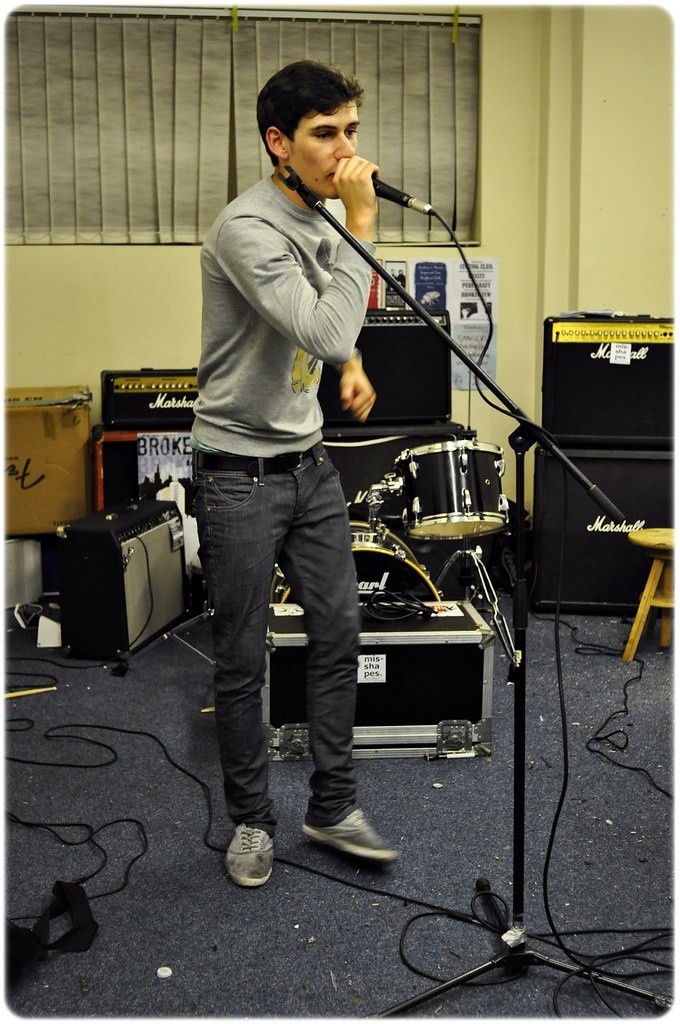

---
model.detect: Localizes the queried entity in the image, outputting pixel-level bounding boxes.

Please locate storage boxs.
[6,385,92,536]
[260,601,495,762]
[530,445,672,615]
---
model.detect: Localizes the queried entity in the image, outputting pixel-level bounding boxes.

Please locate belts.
[193,441,325,473]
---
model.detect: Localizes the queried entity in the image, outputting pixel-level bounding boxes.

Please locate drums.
[271,519,443,604]
[393,438,507,539]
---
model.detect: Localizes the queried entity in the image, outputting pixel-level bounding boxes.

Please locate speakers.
[317,306,465,527]
[53,425,202,659]
[526,316,676,617]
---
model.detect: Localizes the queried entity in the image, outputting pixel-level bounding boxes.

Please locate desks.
[323,420,464,513]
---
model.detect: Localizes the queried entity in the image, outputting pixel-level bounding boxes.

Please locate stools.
[621,528,674,663]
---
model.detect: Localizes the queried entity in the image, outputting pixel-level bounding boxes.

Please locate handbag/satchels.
[7,880,99,989]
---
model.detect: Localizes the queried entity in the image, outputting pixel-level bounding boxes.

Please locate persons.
[188,57,400,889]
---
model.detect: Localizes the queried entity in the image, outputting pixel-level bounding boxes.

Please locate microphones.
[371,176,437,216]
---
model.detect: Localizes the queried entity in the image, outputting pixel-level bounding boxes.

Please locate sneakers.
[303,808,399,862]
[226,824,274,888]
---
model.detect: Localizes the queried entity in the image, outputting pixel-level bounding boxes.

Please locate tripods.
[276,163,673,1020]
